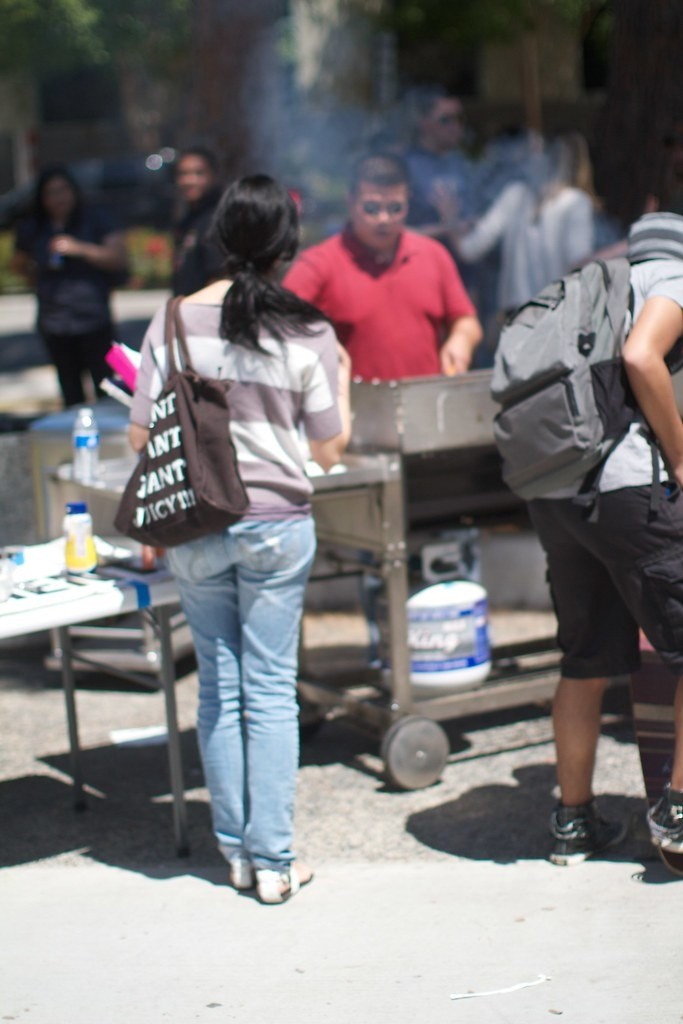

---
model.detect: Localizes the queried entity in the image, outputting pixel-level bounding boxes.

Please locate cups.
[0,557,21,605]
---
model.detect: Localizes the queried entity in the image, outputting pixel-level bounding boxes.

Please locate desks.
[0,536,192,860]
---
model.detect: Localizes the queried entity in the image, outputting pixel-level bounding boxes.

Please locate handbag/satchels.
[112,295,249,547]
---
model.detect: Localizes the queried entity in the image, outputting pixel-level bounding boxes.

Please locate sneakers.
[229,857,254,890]
[646,785,683,854]
[255,858,313,904]
[547,796,626,865]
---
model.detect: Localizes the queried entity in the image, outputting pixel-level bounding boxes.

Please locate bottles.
[61,500,98,572]
[72,407,101,483]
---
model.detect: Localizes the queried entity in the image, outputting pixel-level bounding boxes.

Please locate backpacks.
[489,251,683,501]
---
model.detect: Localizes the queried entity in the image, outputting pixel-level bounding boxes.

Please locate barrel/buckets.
[356,519,492,698]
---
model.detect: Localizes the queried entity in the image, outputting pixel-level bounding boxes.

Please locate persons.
[164,146,233,298]
[128,172,354,907]
[523,210,683,868]
[10,169,130,410]
[351,81,661,370]
[280,151,484,379]
